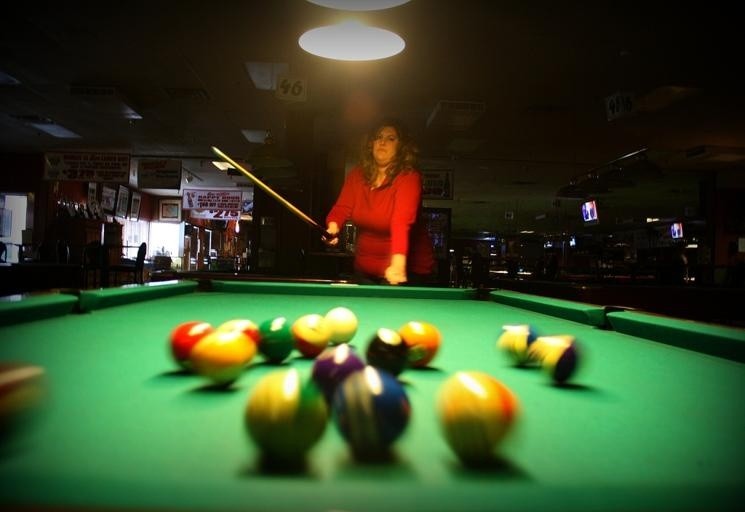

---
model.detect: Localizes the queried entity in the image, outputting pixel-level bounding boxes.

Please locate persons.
[319,114,433,284]
[673,224,681,237]
[585,201,596,221]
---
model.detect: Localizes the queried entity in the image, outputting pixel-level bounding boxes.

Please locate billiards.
[367,321,441,373]
[169,306,364,386]
[244,371,410,456]
[497,322,577,381]
[434,372,515,454]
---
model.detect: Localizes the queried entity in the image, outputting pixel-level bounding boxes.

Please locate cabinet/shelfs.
[51,216,124,288]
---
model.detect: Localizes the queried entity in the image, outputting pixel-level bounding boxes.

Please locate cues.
[212,145,333,241]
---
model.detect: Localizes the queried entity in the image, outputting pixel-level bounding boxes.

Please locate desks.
[0,273,745,512]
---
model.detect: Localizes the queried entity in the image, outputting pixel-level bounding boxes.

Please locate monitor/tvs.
[670,222,683,238]
[579,200,601,227]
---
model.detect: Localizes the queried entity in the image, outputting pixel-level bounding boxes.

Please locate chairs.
[93,243,146,288]
